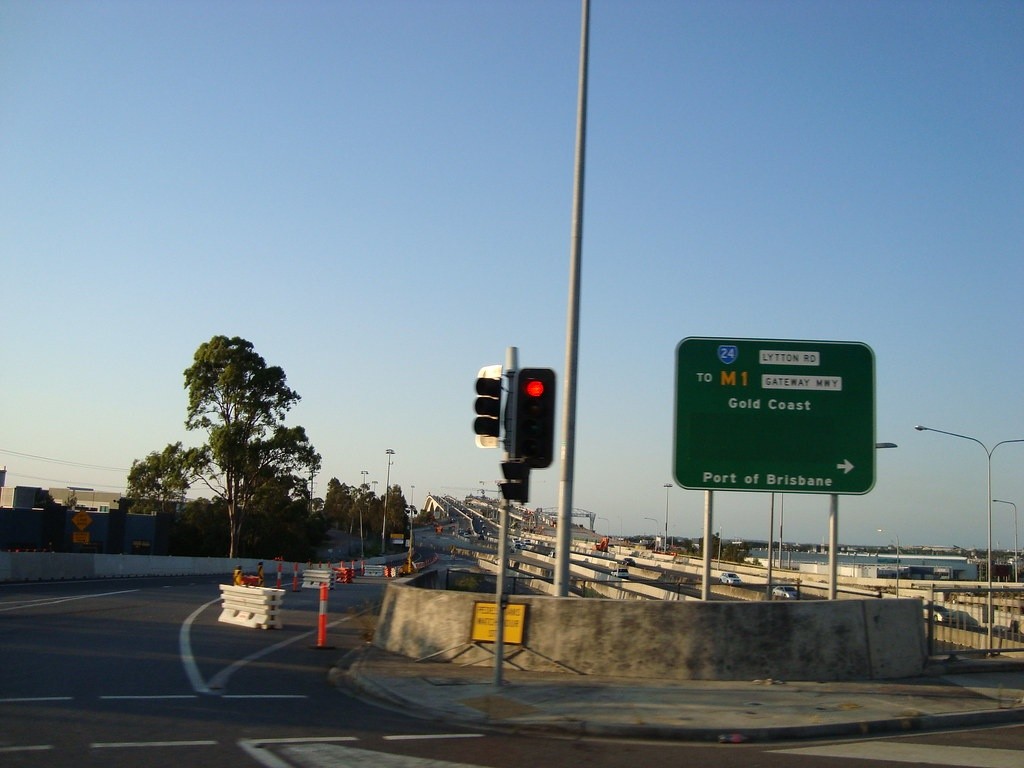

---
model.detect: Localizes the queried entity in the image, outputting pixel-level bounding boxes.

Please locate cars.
[772,586,804,600]
[923,604,956,623]
[718,572,742,586]
[549,551,555,558]
[620,558,635,566]
[458,520,531,550]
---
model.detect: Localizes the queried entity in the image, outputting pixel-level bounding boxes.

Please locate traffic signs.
[672,336,877,495]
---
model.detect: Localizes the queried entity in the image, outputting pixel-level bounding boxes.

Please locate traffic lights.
[472,365,503,448]
[510,367,556,470]
[498,458,530,504]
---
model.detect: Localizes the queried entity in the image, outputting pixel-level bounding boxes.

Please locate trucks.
[611,568,629,579]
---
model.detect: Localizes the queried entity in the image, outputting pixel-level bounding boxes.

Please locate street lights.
[663,483,673,554]
[827,441,899,599]
[361,470,369,493]
[404,503,418,575]
[644,517,659,551]
[597,517,609,540]
[371,480,379,493]
[992,499,1018,582]
[410,485,415,505]
[381,448,396,554]
[913,425,1024,657]
[877,530,900,599]
